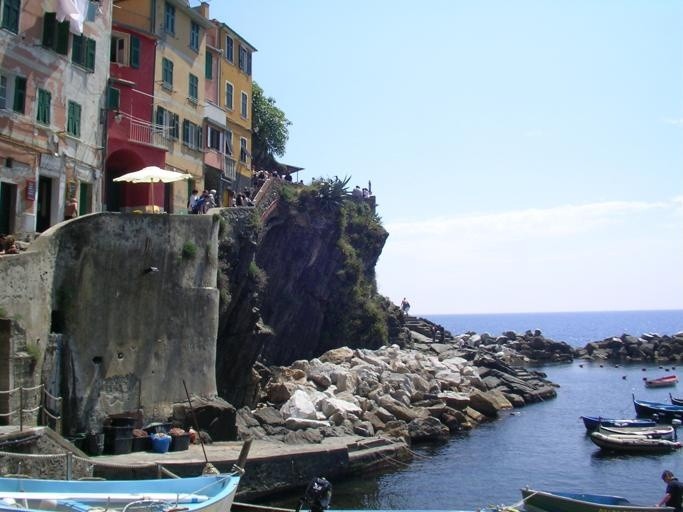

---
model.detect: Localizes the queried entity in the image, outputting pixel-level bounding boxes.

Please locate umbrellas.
[112,165,192,213]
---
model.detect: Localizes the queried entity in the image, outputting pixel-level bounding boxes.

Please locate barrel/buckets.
[85,433,104,456]
[149,432,172,453]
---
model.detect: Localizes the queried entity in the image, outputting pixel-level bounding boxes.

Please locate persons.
[0,237,6,255]
[401,297,410,316]
[431,324,437,341]
[5,236,19,254]
[437,325,444,343]
[187,165,369,214]
[63,198,78,220]
[654,470,682,510]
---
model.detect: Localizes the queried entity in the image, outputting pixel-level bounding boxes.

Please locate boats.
[642,375,678,388]
[579,388,683,454]
[0,433,255,511]
[483,486,679,512]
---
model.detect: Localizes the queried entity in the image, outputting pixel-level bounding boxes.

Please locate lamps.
[100,104,123,125]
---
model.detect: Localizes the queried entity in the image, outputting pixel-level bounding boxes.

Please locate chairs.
[145,205,158,214]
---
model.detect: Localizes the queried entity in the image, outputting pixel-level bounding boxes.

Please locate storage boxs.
[103,414,191,452]
